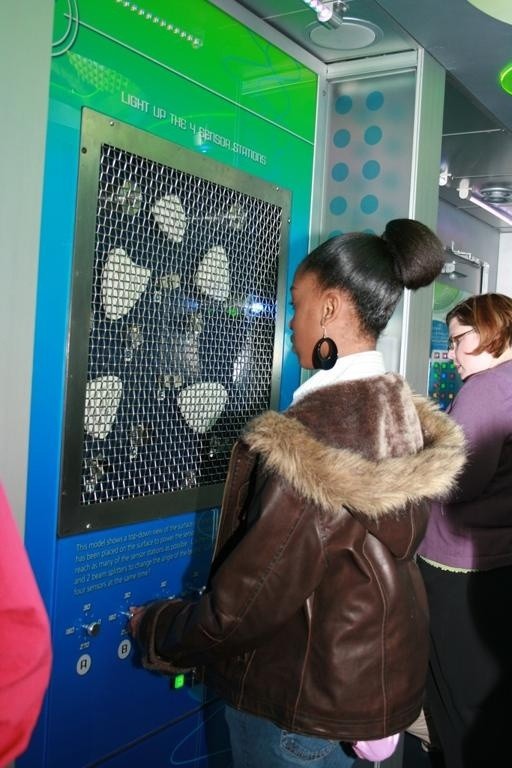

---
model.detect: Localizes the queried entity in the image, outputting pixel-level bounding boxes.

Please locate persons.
[127,227,467,768]
[411,289,511,768]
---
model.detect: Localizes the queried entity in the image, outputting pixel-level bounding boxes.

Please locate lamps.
[300,0,349,31]
[439,174,452,188]
[457,189,512,225]
